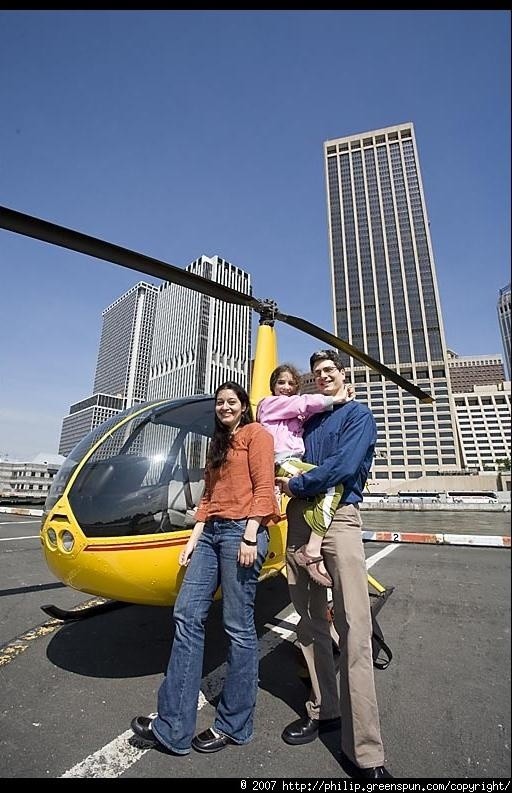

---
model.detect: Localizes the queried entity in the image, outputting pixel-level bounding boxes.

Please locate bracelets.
[240,532,259,546]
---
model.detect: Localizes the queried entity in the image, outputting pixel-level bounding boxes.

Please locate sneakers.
[293,544,333,588]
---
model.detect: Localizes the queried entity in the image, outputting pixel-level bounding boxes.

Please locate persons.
[254,363,345,587]
[280,348,394,778]
[130,379,275,756]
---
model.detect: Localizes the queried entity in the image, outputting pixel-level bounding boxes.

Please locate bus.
[362,490,499,504]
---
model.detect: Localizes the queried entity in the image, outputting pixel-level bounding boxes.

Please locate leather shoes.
[363,766,393,777]
[282,716,341,744]
[191,728,233,753]
[131,716,156,740]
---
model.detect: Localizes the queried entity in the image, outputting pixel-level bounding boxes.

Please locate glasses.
[314,366,337,377]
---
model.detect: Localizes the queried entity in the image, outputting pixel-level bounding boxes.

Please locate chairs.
[153,463,208,528]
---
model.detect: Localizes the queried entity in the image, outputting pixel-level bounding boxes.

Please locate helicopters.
[0,205,435,686]
